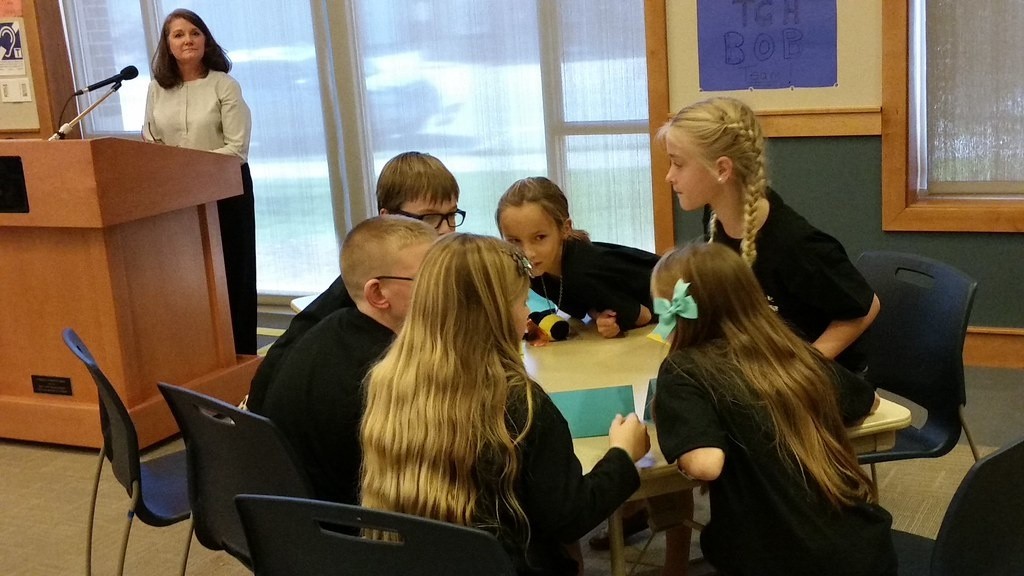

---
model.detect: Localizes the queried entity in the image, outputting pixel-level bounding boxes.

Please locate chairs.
[891,436,1024,576]
[62,328,518,576]
[856,250,981,506]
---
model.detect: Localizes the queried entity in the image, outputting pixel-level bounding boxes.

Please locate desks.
[233,322,913,576]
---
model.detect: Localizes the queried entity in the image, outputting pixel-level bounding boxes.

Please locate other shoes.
[588,506,650,550]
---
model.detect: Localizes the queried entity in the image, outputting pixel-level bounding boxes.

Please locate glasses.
[388,208,466,229]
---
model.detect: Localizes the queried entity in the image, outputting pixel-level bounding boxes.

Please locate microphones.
[75,66,138,96]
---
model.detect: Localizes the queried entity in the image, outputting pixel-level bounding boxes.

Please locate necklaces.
[541,274,563,314]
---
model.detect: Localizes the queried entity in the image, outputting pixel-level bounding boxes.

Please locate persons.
[243,96,899,576]
[140,8,258,355]
